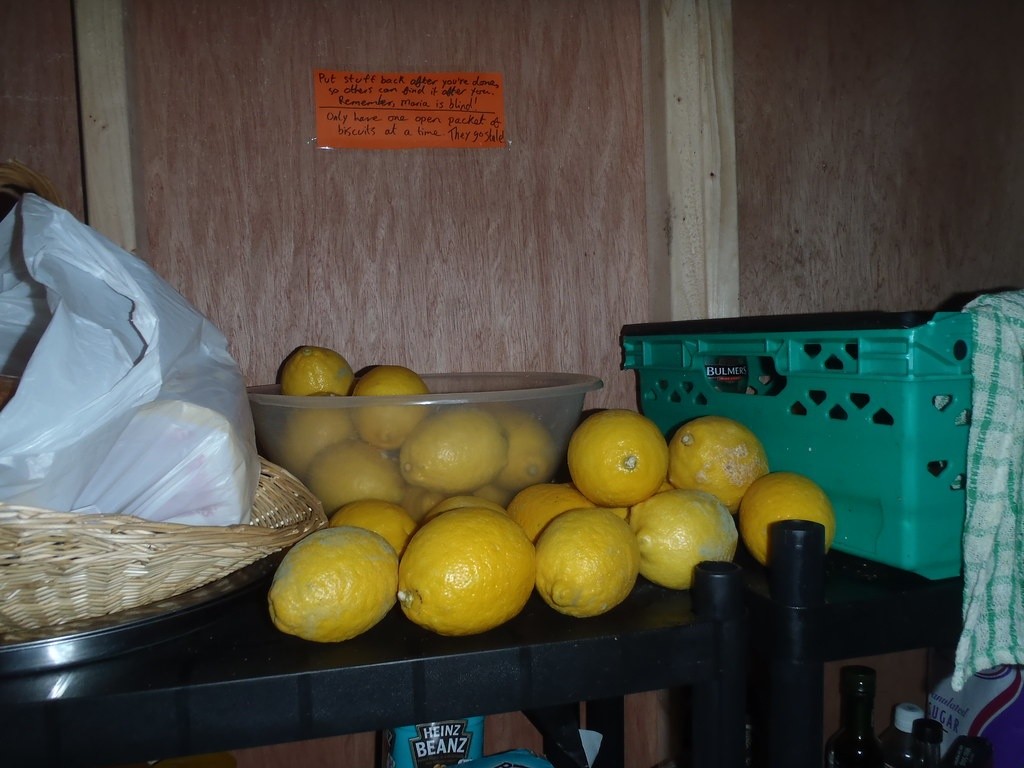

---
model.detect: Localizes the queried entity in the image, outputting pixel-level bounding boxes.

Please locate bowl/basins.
[245,371,603,517]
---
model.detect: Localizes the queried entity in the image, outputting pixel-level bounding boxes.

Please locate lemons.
[268,346,833,643]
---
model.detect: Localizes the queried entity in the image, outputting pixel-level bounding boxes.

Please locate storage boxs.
[619,311,979,580]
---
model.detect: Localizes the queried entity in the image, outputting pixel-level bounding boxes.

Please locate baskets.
[0,459,330,641]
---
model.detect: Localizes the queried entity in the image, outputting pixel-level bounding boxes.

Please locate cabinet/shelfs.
[0,520,964,768]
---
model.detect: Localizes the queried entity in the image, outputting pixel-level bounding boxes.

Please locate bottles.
[687,519,994,768]
